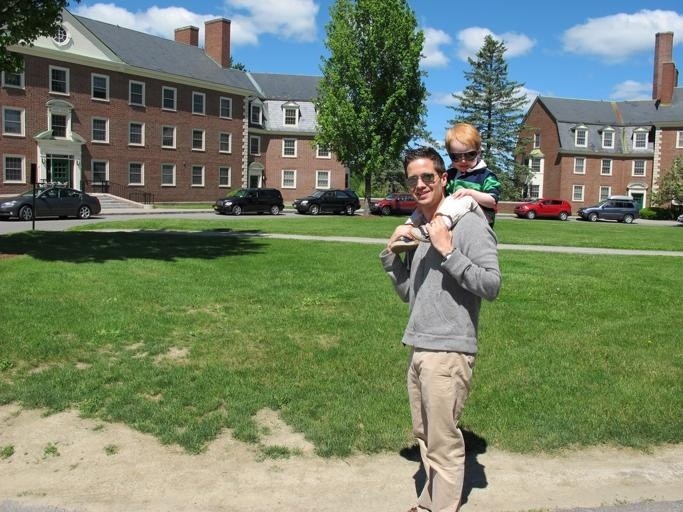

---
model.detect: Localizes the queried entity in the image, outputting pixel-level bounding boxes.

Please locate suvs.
[212,187,285,216]
[291,189,362,215]
[577,194,639,224]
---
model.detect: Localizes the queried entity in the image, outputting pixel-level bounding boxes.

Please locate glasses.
[406,173,439,184]
[449,151,478,162]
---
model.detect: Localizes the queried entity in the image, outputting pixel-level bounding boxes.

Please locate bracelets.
[440,247,457,263]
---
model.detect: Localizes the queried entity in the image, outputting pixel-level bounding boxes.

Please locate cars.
[0,185,101,221]
[676,214,683,224]
[513,198,573,221]
[369,192,416,216]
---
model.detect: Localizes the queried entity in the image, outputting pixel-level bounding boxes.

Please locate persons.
[377,146,505,512]
[385,121,502,256]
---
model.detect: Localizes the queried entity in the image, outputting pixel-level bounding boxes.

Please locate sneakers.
[411,225,431,243]
[391,236,418,254]
